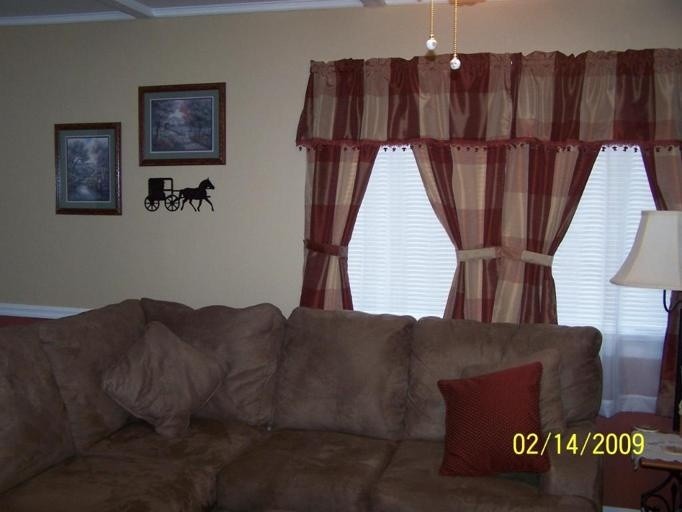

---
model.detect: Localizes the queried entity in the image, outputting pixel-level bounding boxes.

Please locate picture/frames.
[138,80,227,167]
[54,120,123,215]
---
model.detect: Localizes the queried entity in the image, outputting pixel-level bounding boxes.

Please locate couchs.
[2,296,608,512]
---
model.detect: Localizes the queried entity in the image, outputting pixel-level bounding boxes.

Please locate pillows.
[91,317,232,441]
[436,361,551,476]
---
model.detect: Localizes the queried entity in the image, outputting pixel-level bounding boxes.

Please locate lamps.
[609,209,682,511]
